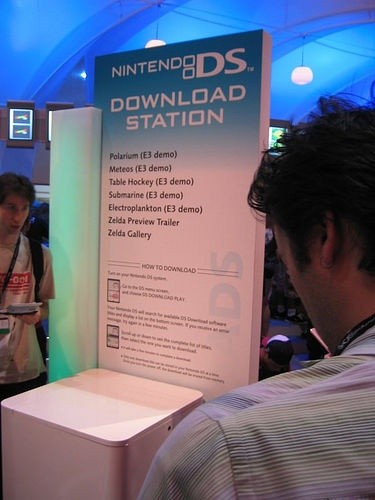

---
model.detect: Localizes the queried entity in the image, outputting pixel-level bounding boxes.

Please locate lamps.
[290,37,314,86]
[144,0,168,49]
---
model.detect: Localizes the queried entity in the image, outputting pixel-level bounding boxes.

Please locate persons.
[260,226,308,375]
[0,172,53,398]
[23,200,53,244]
[135,107,375,500]
